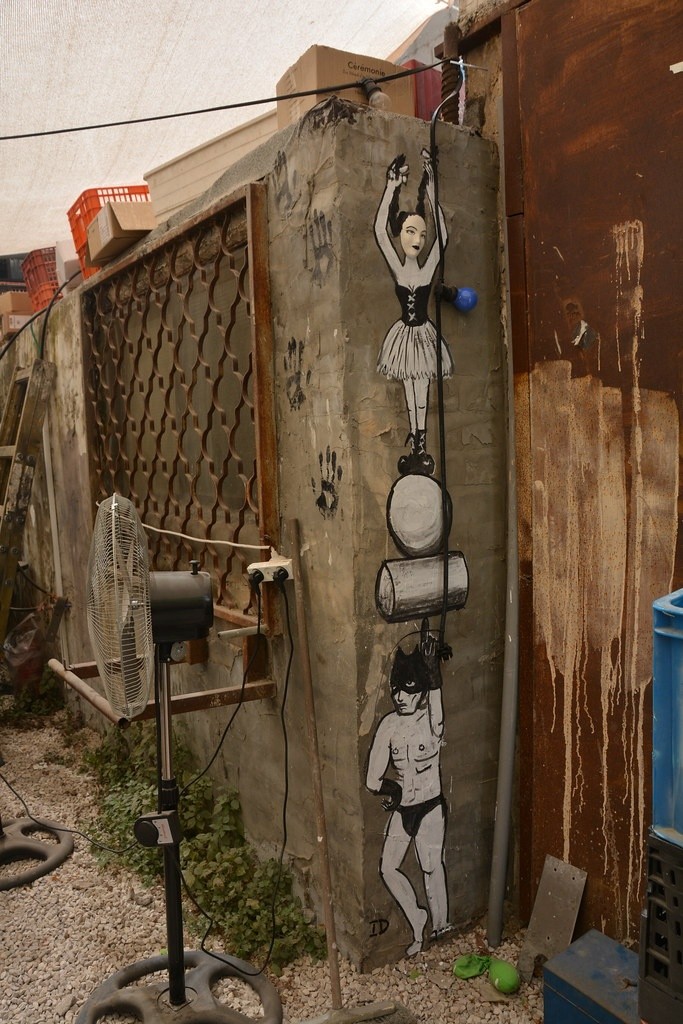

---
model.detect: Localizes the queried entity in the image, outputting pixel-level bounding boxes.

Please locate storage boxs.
[637,589,683,1024]
[0,45,442,349]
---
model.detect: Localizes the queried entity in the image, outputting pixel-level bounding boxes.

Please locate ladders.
[0,358,56,658]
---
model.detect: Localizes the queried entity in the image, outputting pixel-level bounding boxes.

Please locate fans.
[76,492,284,1023]
